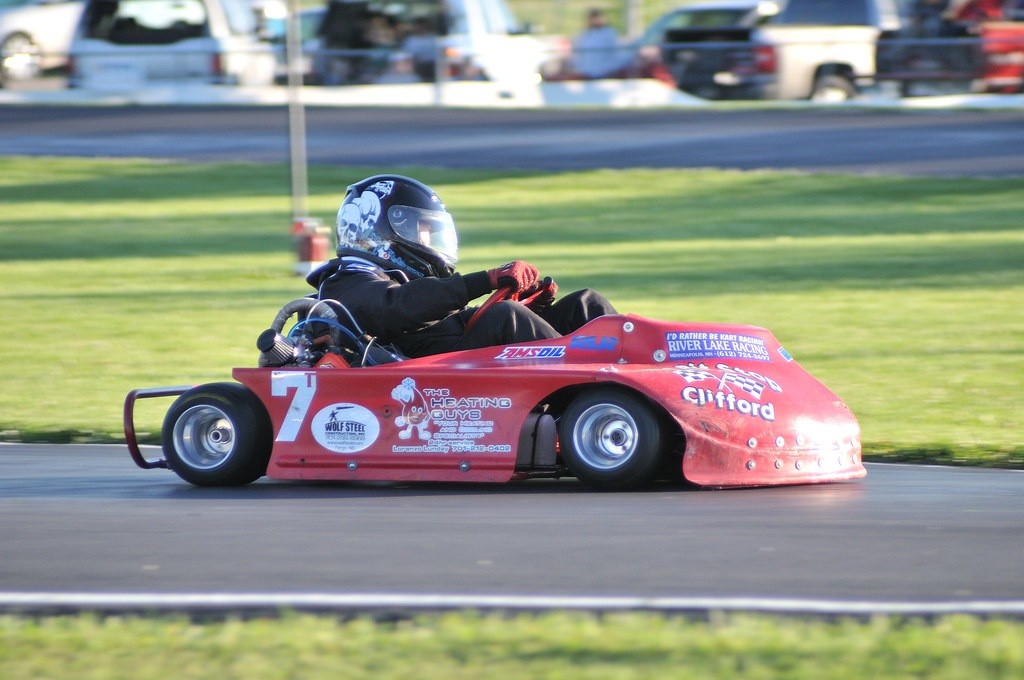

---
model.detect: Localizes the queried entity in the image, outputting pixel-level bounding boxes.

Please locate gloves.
[488,259,558,298]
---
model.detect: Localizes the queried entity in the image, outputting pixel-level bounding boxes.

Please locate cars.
[0,1,558,89]
[873,0,1023,97]
[625,1,901,106]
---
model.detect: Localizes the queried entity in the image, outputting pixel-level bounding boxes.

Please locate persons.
[306,175,620,367]
[567,9,637,78]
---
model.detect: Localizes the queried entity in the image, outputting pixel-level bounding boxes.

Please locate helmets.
[335,174,459,284]
